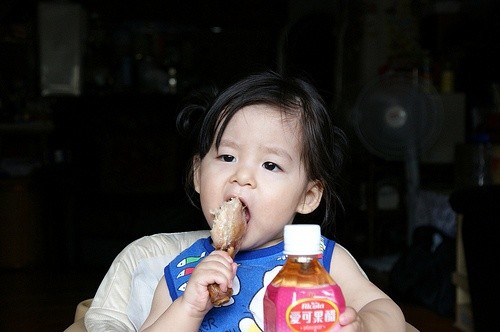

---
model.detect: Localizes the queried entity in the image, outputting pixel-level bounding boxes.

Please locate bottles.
[263,224,346,332]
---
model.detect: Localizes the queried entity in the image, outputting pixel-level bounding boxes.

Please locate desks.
[407,187,465,280]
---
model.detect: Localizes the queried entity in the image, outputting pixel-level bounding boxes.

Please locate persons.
[129,67,407,332]
